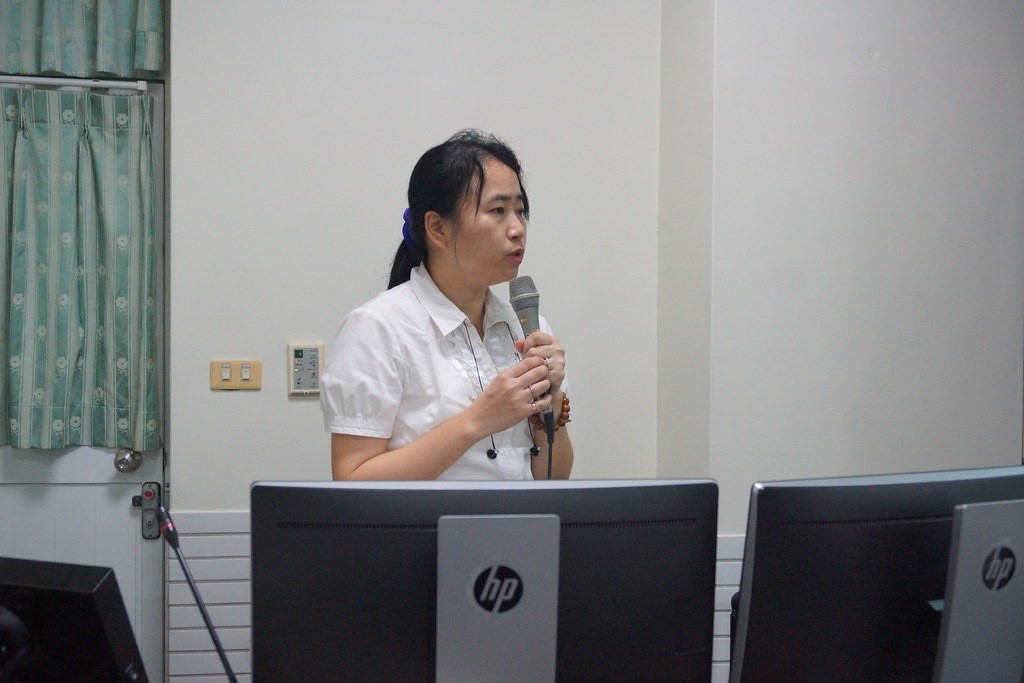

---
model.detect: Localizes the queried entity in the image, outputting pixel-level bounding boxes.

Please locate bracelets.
[530,389,571,434]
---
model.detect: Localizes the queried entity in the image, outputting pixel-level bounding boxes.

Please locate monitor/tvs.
[731,464,1024,683]
[250,478,719,683]
[0,556,149,683]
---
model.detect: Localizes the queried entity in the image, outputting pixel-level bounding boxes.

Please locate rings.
[527,385,536,398]
[532,404,537,414]
[543,357,552,371]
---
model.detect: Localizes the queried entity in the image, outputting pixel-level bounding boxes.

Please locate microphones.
[156,505,237,683]
[509,275,554,444]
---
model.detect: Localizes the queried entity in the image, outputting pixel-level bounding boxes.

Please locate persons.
[314,128,574,481]
